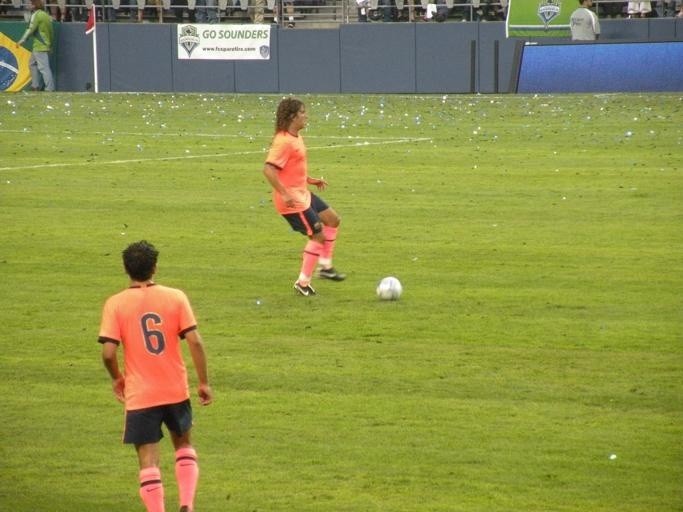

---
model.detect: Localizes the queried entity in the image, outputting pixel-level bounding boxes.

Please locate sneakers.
[320,268,346,281]
[294,281,315,296]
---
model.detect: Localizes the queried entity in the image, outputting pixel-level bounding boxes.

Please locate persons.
[0,0,313,27]
[592,0,683,17]
[16,0,56,92]
[569,0,601,40]
[355,0,509,22]
[97,240,215,512]
[263,98,346,297]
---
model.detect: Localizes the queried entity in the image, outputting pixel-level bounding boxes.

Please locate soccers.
[376,277,402,300]
